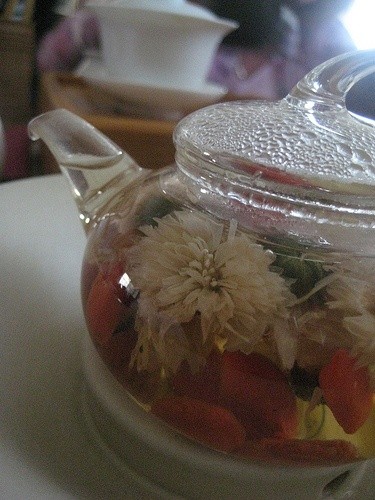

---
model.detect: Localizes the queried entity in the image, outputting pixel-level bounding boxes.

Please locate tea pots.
[27,48,375,499]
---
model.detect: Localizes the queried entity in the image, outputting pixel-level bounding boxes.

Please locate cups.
[84,2,239,109]
